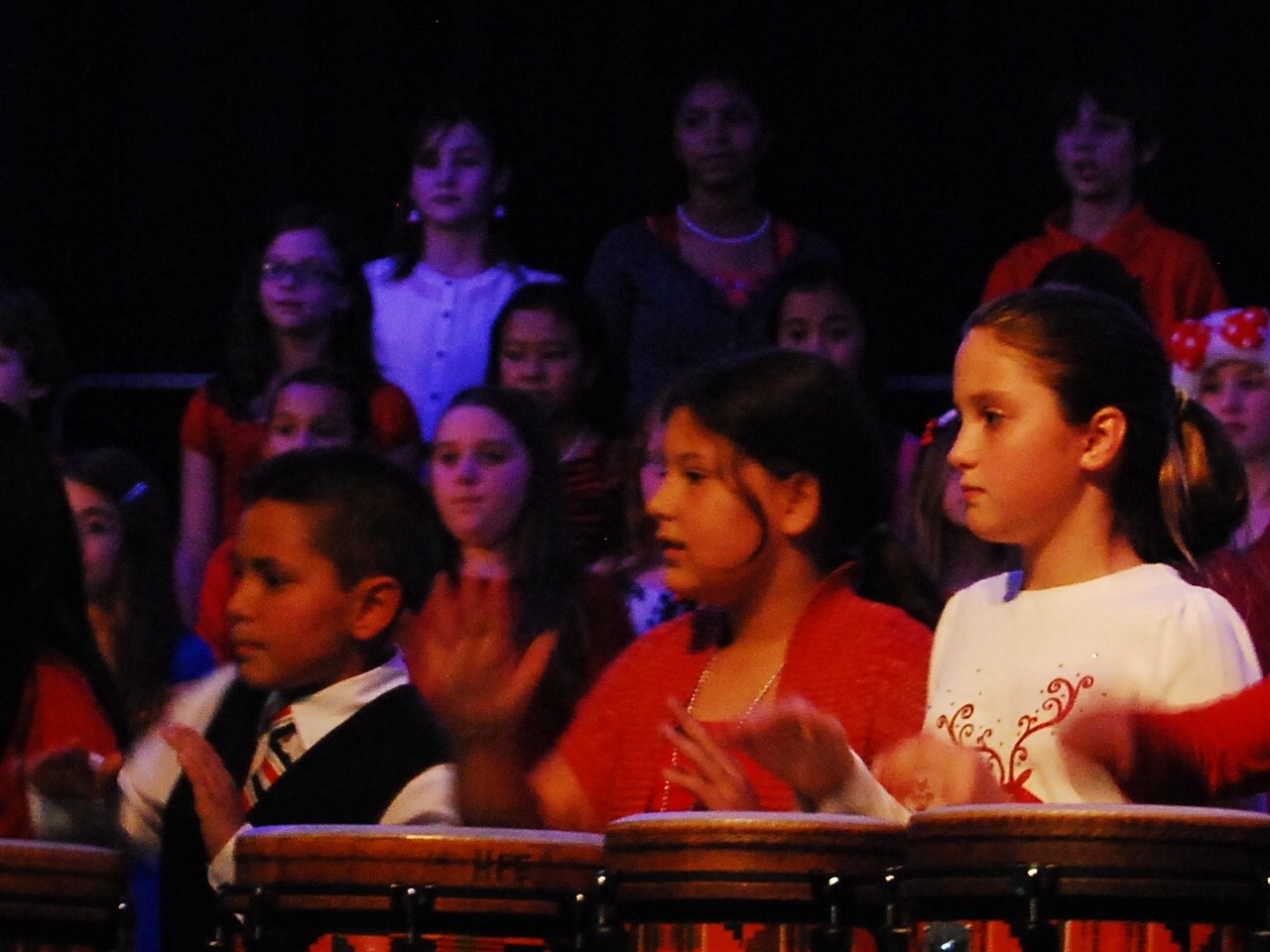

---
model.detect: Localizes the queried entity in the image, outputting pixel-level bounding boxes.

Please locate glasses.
[261,259,346,288]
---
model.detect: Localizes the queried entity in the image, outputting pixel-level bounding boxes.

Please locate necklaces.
[658,650,786,816]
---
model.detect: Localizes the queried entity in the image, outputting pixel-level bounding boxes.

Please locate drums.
[890,803,1269,952]
[227,821,606,952]
[1,836,130,952]
[601,807,894,951]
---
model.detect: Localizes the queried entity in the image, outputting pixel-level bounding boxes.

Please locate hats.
[1169,307,1270,403]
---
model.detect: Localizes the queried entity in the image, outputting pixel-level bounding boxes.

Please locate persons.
[0,59,1270,952]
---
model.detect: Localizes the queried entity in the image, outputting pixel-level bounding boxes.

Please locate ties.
[240,706,300,812]
